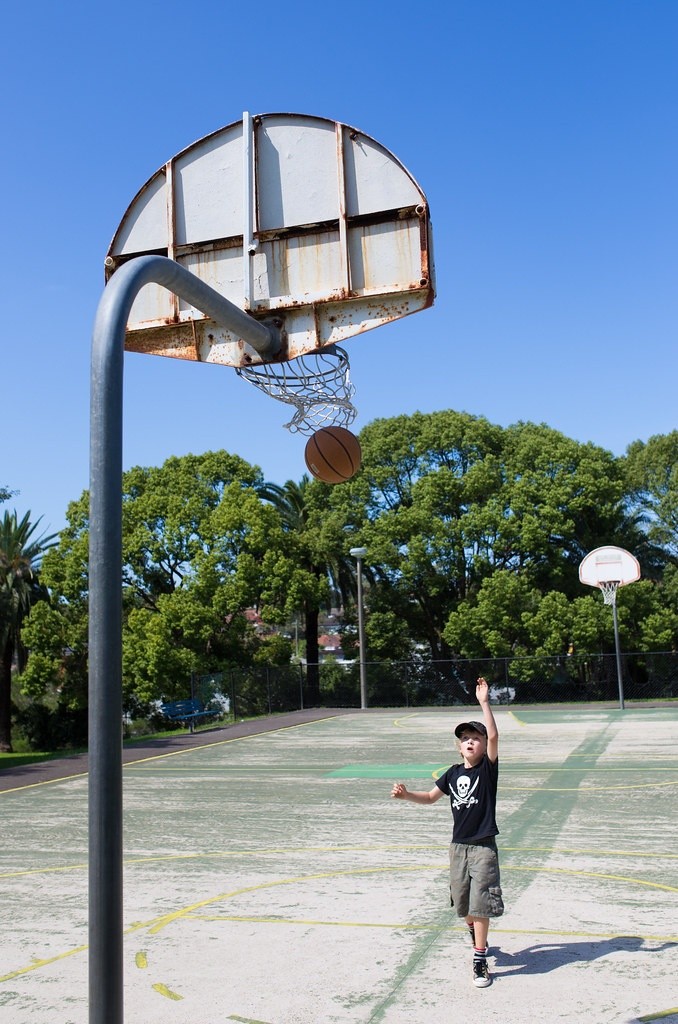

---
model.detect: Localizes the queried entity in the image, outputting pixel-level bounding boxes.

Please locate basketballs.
[304,424,362,485]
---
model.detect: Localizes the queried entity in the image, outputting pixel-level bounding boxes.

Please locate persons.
[391,677,505,988]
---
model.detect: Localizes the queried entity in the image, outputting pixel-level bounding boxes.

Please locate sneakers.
[470,929,489,955]
[472,953,491,987]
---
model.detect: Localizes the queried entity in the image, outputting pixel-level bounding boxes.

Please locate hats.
[455,721,488,739]
